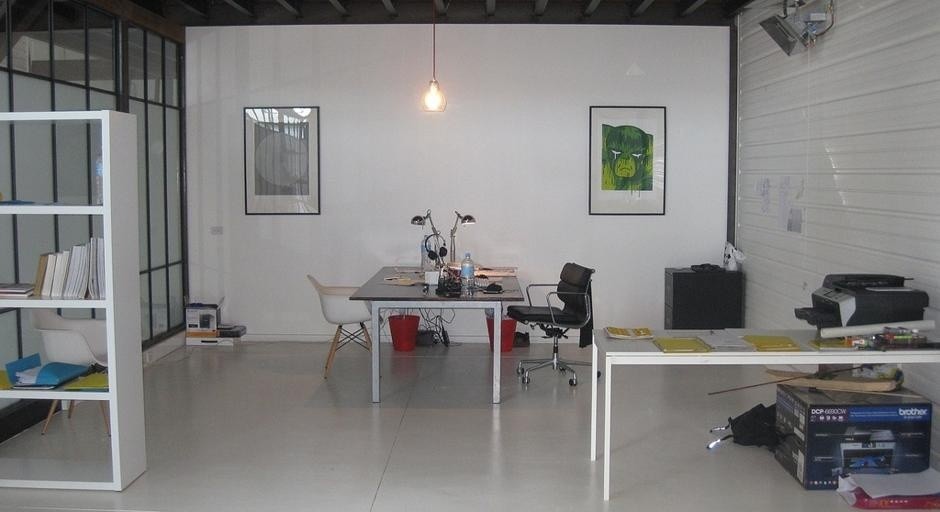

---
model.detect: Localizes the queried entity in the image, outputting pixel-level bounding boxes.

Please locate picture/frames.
[244,106,322,216]
[588,105,667,216]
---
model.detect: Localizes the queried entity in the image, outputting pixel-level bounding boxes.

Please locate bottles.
[420,235,431,270]
[460,253,475,296]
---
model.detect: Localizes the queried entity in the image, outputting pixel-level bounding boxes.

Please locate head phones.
[425,234,447,260]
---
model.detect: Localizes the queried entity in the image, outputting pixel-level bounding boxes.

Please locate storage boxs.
[184,296,224,332]
[774,382,932,490]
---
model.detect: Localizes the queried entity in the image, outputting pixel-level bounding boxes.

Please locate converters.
[442,331,450,346]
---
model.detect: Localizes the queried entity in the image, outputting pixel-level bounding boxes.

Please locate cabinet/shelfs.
[662,268,743,329]
[0,110,144,491]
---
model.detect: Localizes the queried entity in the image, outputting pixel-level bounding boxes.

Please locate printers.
[795,274,929,338]
[839,426,896,473]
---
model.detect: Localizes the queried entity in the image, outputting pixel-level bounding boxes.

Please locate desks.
[588,324,940,500]
[348,265,523,405]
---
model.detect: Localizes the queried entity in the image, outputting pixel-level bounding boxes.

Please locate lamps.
[422,1,448,112]
[410,209,446,265]
[450,210,476,261]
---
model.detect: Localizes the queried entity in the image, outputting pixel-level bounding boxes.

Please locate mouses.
[486,283,502,291]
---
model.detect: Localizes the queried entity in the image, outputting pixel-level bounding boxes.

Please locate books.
[653,331,871,352]
[0,235,105,301]
[605,326,653,341]
[475,267,519,277]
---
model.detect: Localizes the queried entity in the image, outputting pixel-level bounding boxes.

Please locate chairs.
[507,261,601,387]
[305,275,377,379]
[29,308,110,439]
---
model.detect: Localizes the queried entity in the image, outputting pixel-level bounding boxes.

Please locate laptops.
[436,251,490,288]
